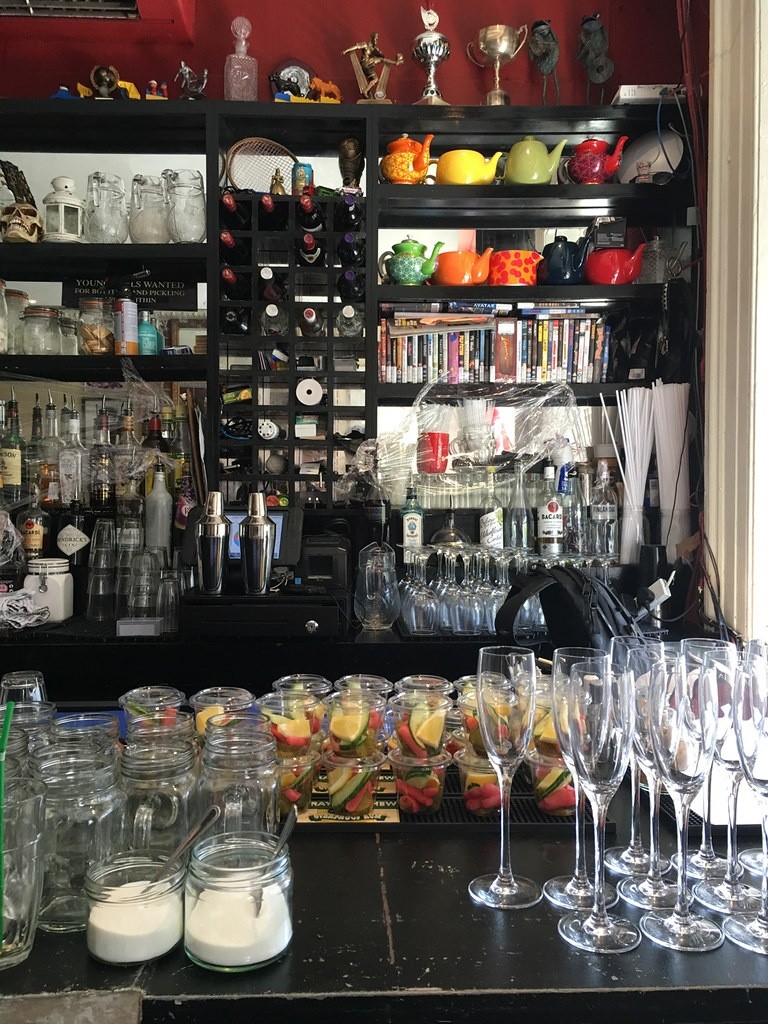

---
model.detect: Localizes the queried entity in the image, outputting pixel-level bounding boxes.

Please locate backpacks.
[495,557,653,673]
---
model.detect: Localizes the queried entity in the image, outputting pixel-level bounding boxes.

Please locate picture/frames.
[83,396,122,448]
[171,318,207,354]
[172,382,207,406]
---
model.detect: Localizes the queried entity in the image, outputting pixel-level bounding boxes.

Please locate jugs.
[83,168,205,244]
[354,542,401,630]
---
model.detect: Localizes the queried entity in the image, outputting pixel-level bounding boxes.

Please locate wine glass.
[468,637,768,956]
[396,544,620,633]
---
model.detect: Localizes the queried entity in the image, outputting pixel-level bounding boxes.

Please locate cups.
[81,849,190,966]
[183,830,296,973]
[0,777,48,972]
[260,674,577,819]
[0,671,281,867]
[85,519,180,633]
[27,752,130,934]
[374,423,661,514]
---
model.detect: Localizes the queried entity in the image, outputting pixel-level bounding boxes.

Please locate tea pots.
[376,132,688,287]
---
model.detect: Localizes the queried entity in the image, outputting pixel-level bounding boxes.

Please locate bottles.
[399,458,696,572]
[219,138,368,338]
[0,280,207,566]
[173,491,277,598]
[225,16,259,102]
[22,558,75,622]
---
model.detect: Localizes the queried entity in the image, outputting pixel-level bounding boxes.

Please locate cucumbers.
[285,732,567,810]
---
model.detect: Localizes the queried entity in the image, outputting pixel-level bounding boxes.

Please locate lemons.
[195,702,574,794]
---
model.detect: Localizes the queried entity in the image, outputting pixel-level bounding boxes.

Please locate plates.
[617,131,683,183]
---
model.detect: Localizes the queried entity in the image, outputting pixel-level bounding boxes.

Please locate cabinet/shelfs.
[0,95,692,681]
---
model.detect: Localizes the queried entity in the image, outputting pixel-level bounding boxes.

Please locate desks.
[0,763,768,1024]
